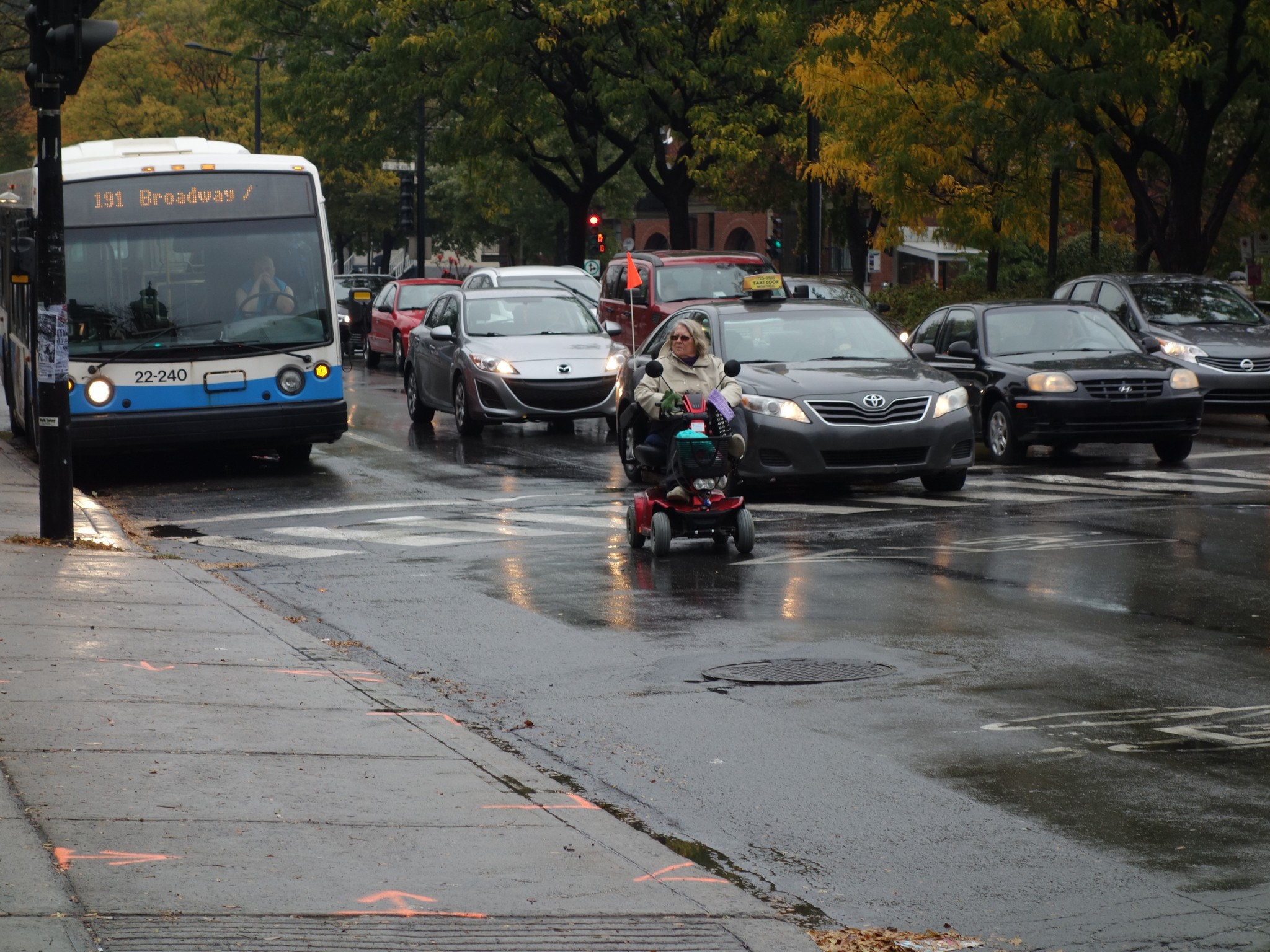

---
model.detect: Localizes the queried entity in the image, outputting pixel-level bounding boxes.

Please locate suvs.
[1050,271,1270,419]
[598,247,797,354]
[459,264,603,327]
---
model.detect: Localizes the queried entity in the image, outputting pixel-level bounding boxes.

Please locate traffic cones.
[626,251,643,290]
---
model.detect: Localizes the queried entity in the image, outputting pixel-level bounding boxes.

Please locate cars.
[902,300,1203,466]
[364,277,464,370]
[403,289,631,435]
[333,273,398,356]
[613,273,975,496]
[783,276,910,342]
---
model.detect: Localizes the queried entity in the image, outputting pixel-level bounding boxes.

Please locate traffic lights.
[587,214,600,256]
[764,238,782,260]
[44,0,120,95]
[597,232,606,253]
[879,220,894,257]
[771,218,784,254]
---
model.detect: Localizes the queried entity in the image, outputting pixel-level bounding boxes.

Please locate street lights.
[409,4,530,277]
[183,43,334,154]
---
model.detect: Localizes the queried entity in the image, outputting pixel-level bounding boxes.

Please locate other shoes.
[666,486,690,503]
[729,434,746,460]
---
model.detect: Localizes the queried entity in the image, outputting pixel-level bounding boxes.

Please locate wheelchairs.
[624,358,756,559]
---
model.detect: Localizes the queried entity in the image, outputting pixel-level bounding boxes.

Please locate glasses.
[670,335,692,341]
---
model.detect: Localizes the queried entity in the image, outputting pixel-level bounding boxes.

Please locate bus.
[0,134,349,477]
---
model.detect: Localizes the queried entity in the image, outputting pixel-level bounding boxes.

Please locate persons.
[634,319,747,501]
[236,255,296,318]
